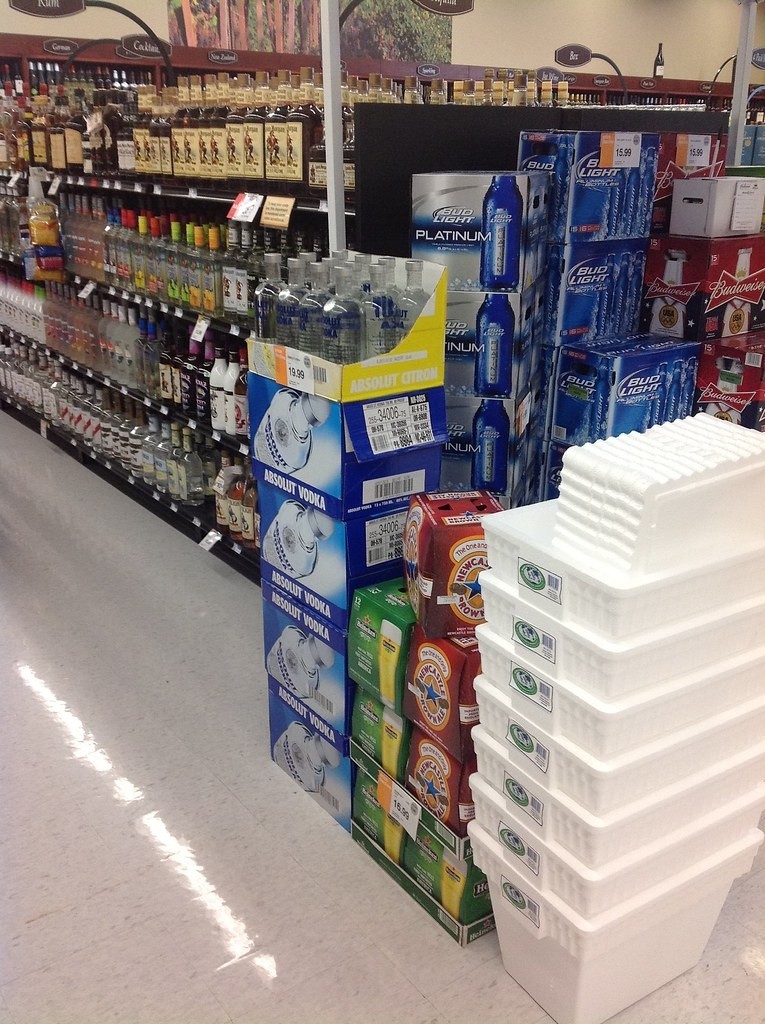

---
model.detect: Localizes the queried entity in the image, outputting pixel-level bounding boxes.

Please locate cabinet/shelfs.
[0,34,765,588]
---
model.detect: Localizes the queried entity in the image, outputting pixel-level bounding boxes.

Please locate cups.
[590,356,610,440]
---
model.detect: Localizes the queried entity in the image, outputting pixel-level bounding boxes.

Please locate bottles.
[0,263,260,555]
[604,147,656,237]
[264,499,334,579]
[598,251,644,334]
[482,173,524,291]
[568,92,719,113]
[653,42,665,79]
[650,357,696,426]
[1,190,330,328]
[474,293,515,399]
[704,356,742,425]
[273,720,341,790]
[266,625,335,697]
[0,58,567,200]
[255,388,330,474]
[257,249,434,362]
[723,99,765,125]
[647,248,687,339]
[472,400,510,494]
[722,248,751,335]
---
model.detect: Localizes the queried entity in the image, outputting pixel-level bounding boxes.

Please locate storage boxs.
[241,123,765,1024]
